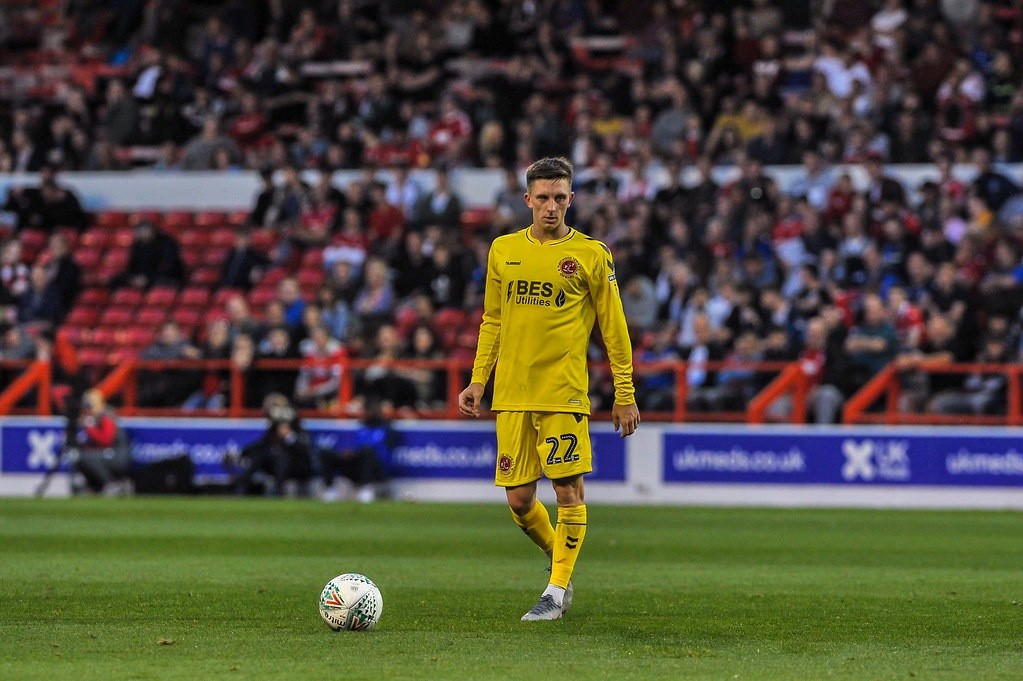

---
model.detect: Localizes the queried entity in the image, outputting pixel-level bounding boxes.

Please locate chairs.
[20,212,324,374]
[396,310,489,348]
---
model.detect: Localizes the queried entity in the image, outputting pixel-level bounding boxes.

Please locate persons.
[64,395,133,496]
[0,0,1023,395]
[229,392,310,500]
[320,401,400,506]
[458,156,641,621]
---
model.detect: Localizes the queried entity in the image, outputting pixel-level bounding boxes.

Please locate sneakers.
[521,594,563,621]
[546,565,573,611]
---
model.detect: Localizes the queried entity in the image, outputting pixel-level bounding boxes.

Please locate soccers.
[318,573,384,632]
